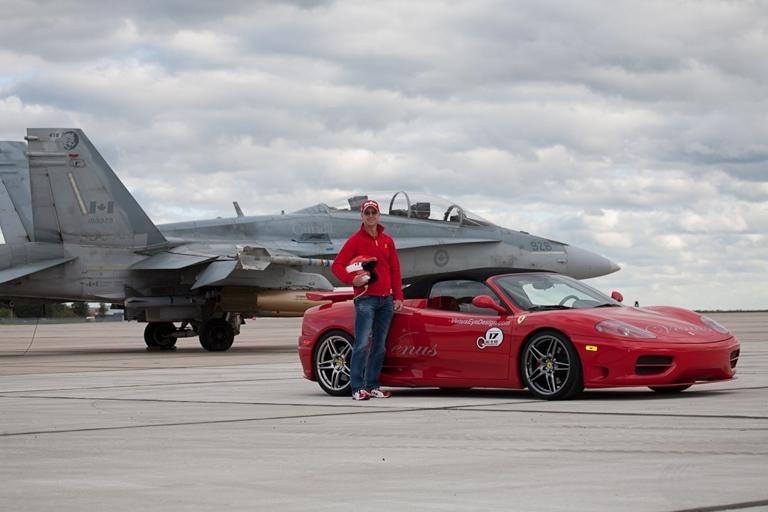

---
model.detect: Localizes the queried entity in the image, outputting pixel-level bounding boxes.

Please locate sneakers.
[352,390,369,400]
[366,388,392,398]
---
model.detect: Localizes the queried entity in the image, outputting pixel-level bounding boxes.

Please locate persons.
[330,200,404,402]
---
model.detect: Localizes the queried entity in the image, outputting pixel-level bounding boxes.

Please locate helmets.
[346,256,378,287]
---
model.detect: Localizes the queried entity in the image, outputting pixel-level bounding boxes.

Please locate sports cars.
[298,268,740,398]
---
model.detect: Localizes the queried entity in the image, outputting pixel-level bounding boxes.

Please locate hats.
[360,200,379,214]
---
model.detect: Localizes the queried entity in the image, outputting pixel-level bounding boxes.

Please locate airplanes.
[1,126,622,352]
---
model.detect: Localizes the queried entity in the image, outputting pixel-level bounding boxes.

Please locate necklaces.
[367,228,379,236]
